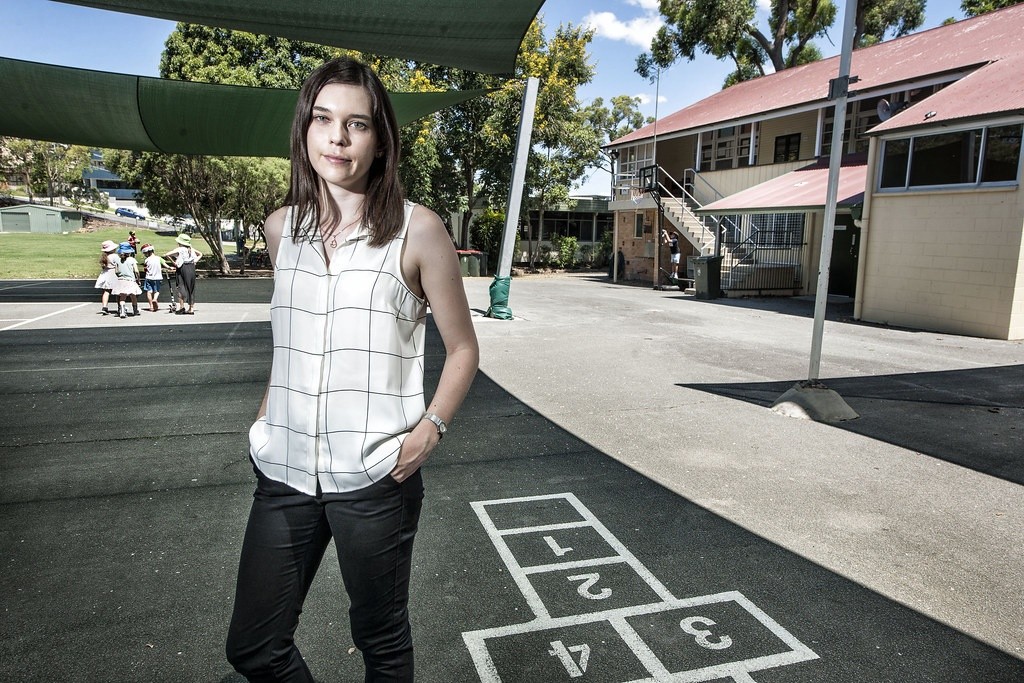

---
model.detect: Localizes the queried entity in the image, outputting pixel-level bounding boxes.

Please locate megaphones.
[877,99,909,121]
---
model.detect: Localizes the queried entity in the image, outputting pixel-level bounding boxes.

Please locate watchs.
[422,412,447,441]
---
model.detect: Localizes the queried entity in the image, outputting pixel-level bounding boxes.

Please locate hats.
[101,240,118,252]
[141,243,154,252]
[175,234,191,247]
[118,242,135,253]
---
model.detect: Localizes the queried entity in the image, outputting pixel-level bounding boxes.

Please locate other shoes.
[149,308,154,311]
[134,311,140,315]
[176,308,186,314]
[102,309,111,315]
[152,300,158,311]
[120,312,125,317]
[185,310,195,314]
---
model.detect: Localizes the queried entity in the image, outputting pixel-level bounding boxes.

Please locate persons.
[225,56,481,683]
[95,230,203,319]
[662,229,681,279]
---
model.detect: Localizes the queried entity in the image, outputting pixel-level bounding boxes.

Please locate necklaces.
[320,217,361,248]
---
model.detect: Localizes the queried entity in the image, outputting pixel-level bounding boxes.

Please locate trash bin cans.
[468,250,489,277]
[690,255,725,300]
[456,250,471,277]
